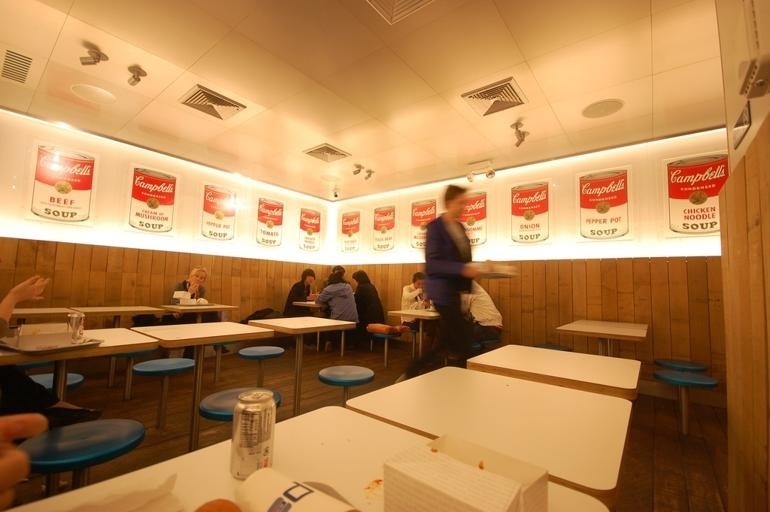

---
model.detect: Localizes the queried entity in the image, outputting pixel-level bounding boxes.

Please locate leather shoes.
[43,407,101,426]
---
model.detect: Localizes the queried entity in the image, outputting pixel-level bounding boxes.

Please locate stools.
[408,329,420,362]
[200,389,283,424]
[533,343,561,351]
[370,339,373,352]
[480,339,499,346]
[373,332,403,368]
[27,372,84,392]
[239,346,285,389]
[652,369,719,436]
[15,418,146,492]
[132,357,196,436]
[469,343,482,351]
[318,365,375,408]
[654,359,710,421]
[341,331,344,357]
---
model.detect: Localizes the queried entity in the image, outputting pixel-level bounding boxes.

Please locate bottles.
[67,313,85,344]
[230,390,276,482]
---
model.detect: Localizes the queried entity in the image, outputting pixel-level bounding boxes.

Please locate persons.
[352,270,384,353]
[314,271,359,354]
[459,278,503,367]
[402,272,438,363]
[0,275,102,471]
[395,185,494,383]
[277,270,319,346]
[320,266,351,297]
[172,267,230,372]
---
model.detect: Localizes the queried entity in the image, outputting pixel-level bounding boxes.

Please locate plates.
[0,331,101,353]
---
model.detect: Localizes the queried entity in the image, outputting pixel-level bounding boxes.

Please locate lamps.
[352,163,364,175]
[511,123,524,141]
[333,188,339,197]
[80,50,108,66]
[465,166,475,184]
[514,131,529,147]
[127,65,148,85]
[364,170,375,181]
[485,163,496,179]
